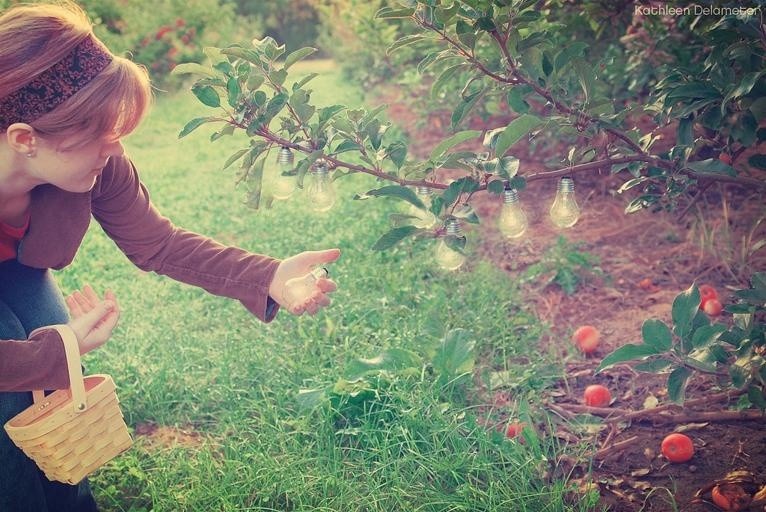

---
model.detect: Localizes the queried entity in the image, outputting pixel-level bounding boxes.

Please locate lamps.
[304,152,337,217]
[550,176,580,231]
[269,142,301,204]
[438,220,465,273]
[281,266,329,308]
[498,187,527,241]
[412,187,437,234]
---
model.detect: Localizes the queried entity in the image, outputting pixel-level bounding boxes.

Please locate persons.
[0,6,342,511]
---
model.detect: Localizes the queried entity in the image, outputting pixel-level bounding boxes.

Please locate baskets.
[3,324,136,487]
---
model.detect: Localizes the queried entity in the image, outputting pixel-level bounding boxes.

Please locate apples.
[583,384,612,410]
[574,324,600,354]
[505,425,530,448]
[695,283,718,310]
[661,432,696,464]
[703,299,724,316]
